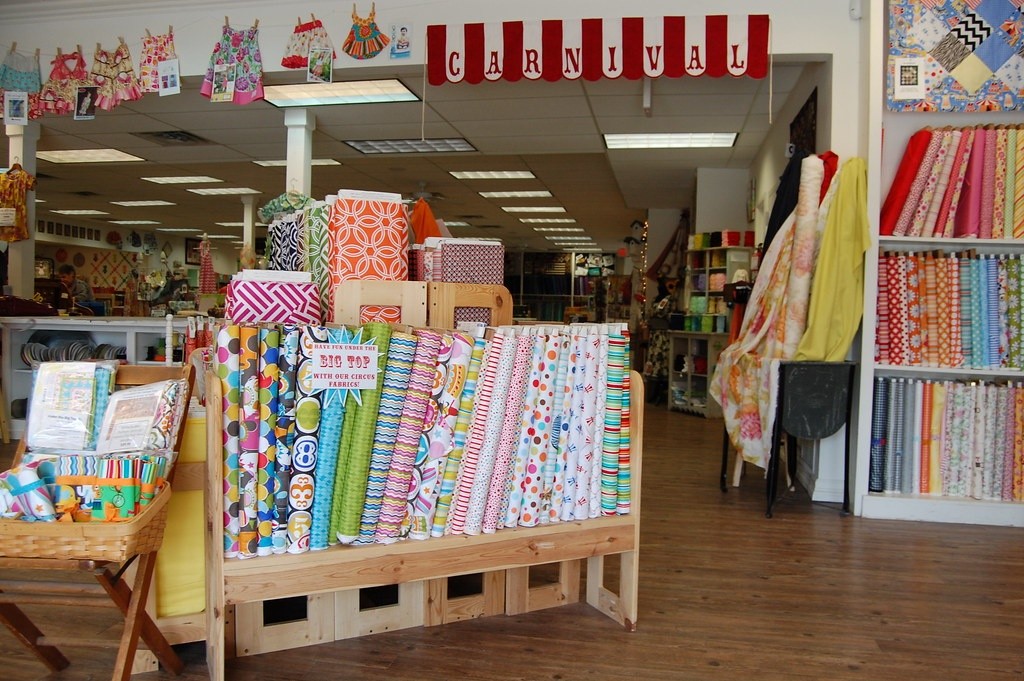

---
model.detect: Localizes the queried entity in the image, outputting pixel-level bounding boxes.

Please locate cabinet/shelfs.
[505,250,613,323]
[0,315,220,440]
[665,245,753,418]
[852,0,1024,529]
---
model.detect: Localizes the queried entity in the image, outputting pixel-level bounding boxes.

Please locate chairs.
[0,357,196,681]
[35,280,94,316]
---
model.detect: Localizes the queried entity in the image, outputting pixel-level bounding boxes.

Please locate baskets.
[0,480,172,562]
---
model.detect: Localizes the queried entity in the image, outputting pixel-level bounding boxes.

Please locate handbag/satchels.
[659,229,684,278]
[0,295,59,316]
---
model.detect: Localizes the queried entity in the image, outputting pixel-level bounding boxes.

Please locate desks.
[720,361,858,518]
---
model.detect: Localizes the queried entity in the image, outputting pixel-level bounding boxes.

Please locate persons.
[59,264,94,303]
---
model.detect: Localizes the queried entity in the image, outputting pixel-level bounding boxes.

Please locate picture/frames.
[185,238,200,266]
